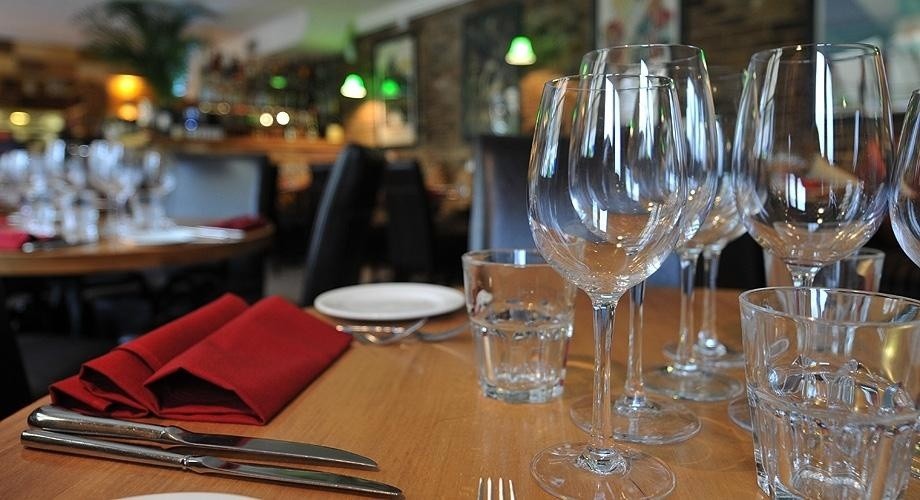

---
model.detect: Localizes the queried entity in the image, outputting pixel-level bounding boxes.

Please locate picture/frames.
[371,30,422,150]
[458,4,522,140]
[588,1,683,53]
[802,0,919,123]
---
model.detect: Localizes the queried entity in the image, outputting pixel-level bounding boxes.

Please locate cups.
[731,280,916,500]
[462,247,571,406]
[758,246,891,293]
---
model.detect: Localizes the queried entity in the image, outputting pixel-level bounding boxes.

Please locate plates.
[312,280,465,322]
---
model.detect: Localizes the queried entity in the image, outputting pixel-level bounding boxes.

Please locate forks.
[331,322,470,347]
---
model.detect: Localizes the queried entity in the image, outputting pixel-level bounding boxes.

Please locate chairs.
[468,130,684,287]
[380,156,444,280]
[295,140,386,305]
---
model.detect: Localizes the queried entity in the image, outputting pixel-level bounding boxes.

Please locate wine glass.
[0,134,177,239]
[527,39,920,499]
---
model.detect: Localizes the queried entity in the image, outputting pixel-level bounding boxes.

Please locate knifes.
[26,408,387,469]
[22,427,402,497]
